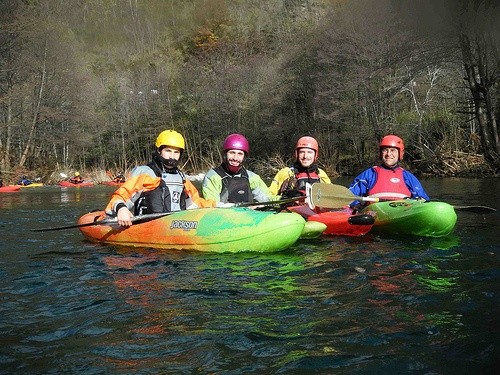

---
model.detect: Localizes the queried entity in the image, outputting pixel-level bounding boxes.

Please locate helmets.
[379,135,404,162]
[295,136,318,162]
[224,134,249,153]
[155,130,185,152]
[74,172,80,176]
[23,175,26,179]
[118,171,122,174]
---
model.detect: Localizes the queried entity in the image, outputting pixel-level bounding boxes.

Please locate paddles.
[450,205,497,214]
[311,183,403,208]
[30,194,307,232]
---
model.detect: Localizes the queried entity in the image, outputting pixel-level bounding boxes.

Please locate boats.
[0,182,46,192]
[77,200,458,252]
[99,181,125,185]
[57,180,95,186]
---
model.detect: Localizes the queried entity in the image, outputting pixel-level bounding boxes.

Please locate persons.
[348,135,430,212]
[268,137,332,215]
[19,176,30,186]
[104,129,218,227]
[70,172,83,183]
[202,134,280,211]
[113,171,125,183]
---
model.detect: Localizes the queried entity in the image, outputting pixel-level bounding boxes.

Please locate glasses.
[381,148,398,154]
